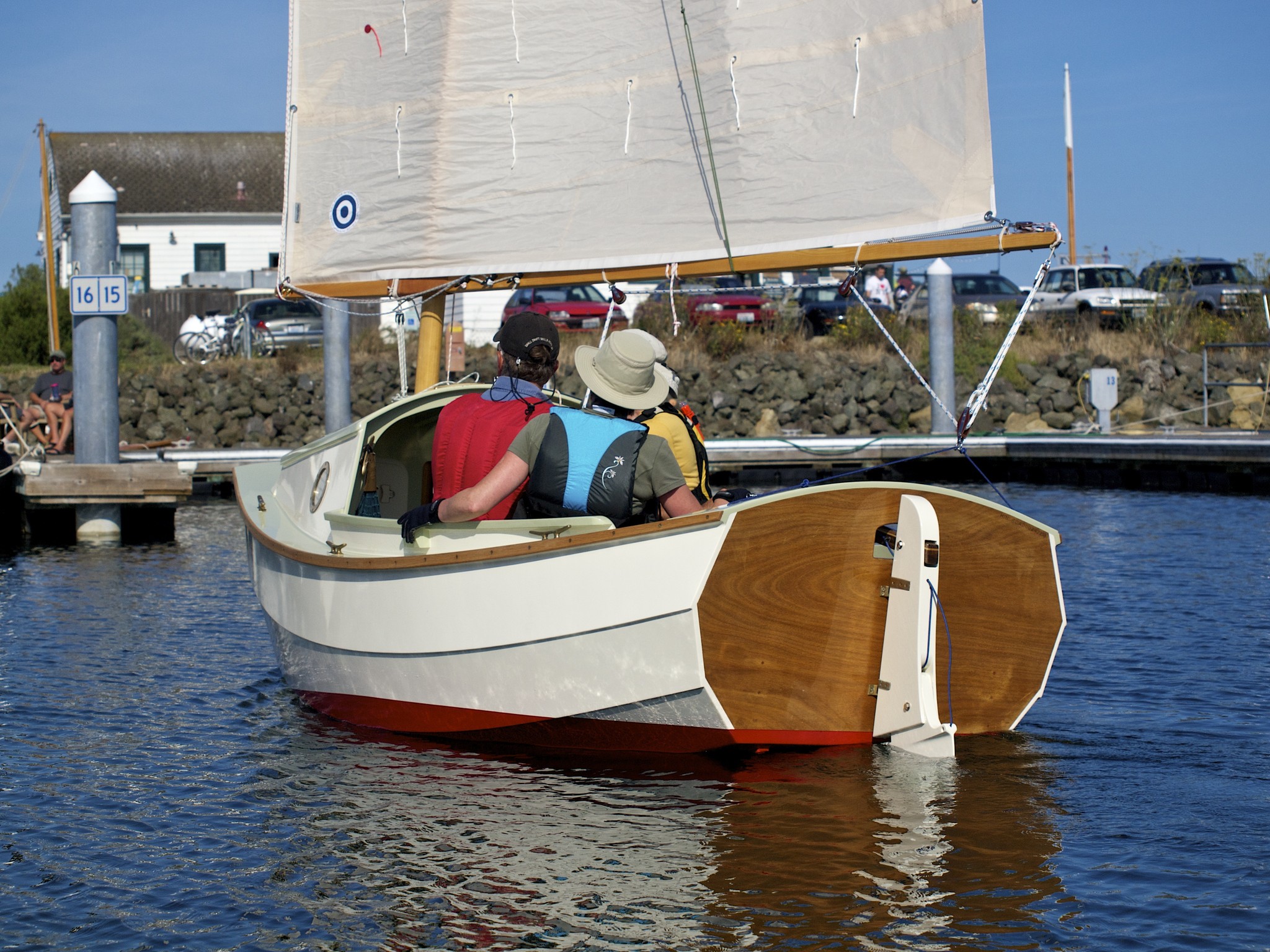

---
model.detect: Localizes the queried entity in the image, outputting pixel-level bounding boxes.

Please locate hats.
[49,350,65,358]
[492,312,561,365]
[575,330,670,410]
[628,327,678,399]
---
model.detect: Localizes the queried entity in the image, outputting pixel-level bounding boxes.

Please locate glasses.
[50,358,61,362]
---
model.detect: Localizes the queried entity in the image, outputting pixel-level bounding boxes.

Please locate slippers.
[43,443,65,455]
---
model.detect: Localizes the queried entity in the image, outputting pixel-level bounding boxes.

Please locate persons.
[0,391,34,451]
[396,328,756,544]
[863,265,897,313]
[619,329,714,520]
[894,284,910,304]
[898,266,915,295]
[26,349,73,457]
[430,312,563,520]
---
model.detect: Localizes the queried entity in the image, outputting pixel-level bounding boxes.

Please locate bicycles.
[172,306,276,365]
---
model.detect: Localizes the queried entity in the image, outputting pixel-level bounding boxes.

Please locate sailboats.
[228,2,1068,767]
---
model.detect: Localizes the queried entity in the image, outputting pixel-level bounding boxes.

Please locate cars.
[235,298,326,356]
[648,277,781,332]
[793,268,1036,334]
[1025,263,1173,328]
[1141,256,1269,317]
[498,284,628,331]
